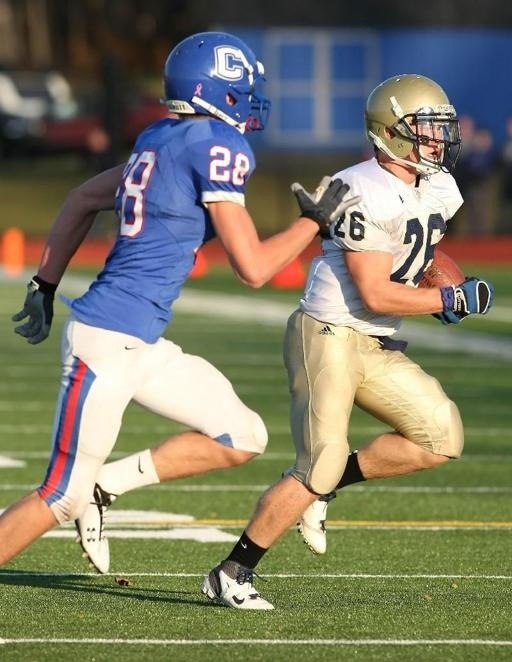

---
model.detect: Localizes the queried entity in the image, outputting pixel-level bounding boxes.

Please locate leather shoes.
[431,279,494,327]
[288,177,361,236]
[12,277,57,346]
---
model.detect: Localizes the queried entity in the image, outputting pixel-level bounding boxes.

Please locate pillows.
[363,73,461,177]
[163,31,270,134]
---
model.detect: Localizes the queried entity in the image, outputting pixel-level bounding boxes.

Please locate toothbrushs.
[420,250,467,289]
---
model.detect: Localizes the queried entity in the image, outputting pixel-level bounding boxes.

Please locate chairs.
[75,482,113,574]
[282,470,338,557]
[202,556,273,612]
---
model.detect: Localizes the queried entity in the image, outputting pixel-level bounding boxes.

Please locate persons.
[198,74,494,612]
[436,113,512,235]
[1,13,178,172]
[0,32,362,572]
